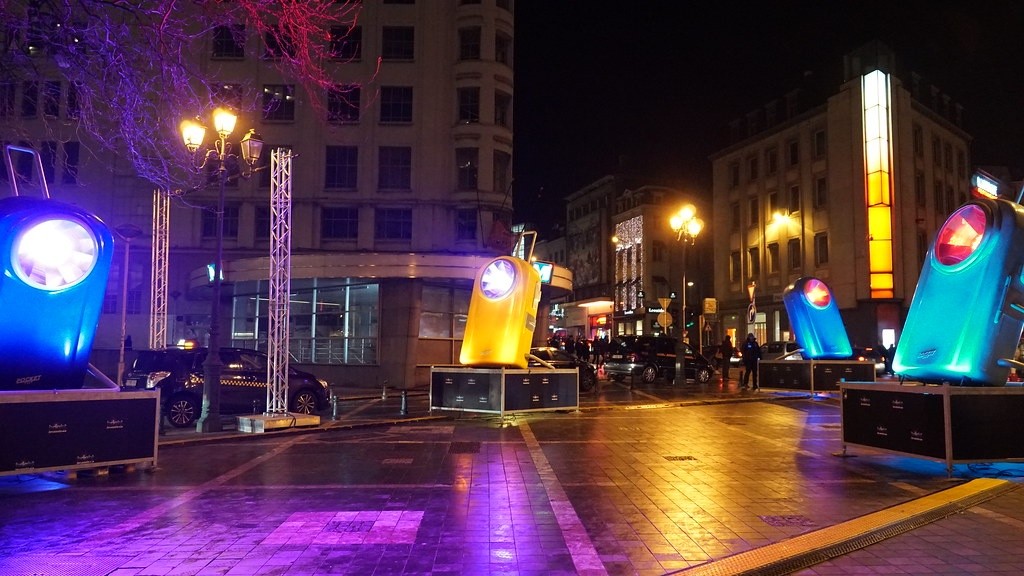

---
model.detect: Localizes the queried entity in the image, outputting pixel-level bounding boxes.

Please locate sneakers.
[753,385,758,390]
[743,385,751,389]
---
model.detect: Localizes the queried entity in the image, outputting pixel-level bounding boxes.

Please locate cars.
[701,346,742,367]
[506,345,597,392]
[848,348,886,377]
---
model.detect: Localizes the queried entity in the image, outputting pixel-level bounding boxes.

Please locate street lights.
[179,106,261,437]
[670,206,701,389]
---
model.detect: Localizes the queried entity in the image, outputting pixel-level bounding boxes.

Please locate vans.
[759,341,803,361]
[604,334,715,384]
[120,346,330,429]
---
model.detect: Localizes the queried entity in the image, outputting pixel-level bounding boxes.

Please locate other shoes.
[723,376,731,381]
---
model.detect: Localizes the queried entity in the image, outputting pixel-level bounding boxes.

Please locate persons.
[720,335,733,380]
[124,335,132,351]
[1016,342,1024,382]
[740,333,762,389]
[873,340,896,377]
[546,333,610,365]
